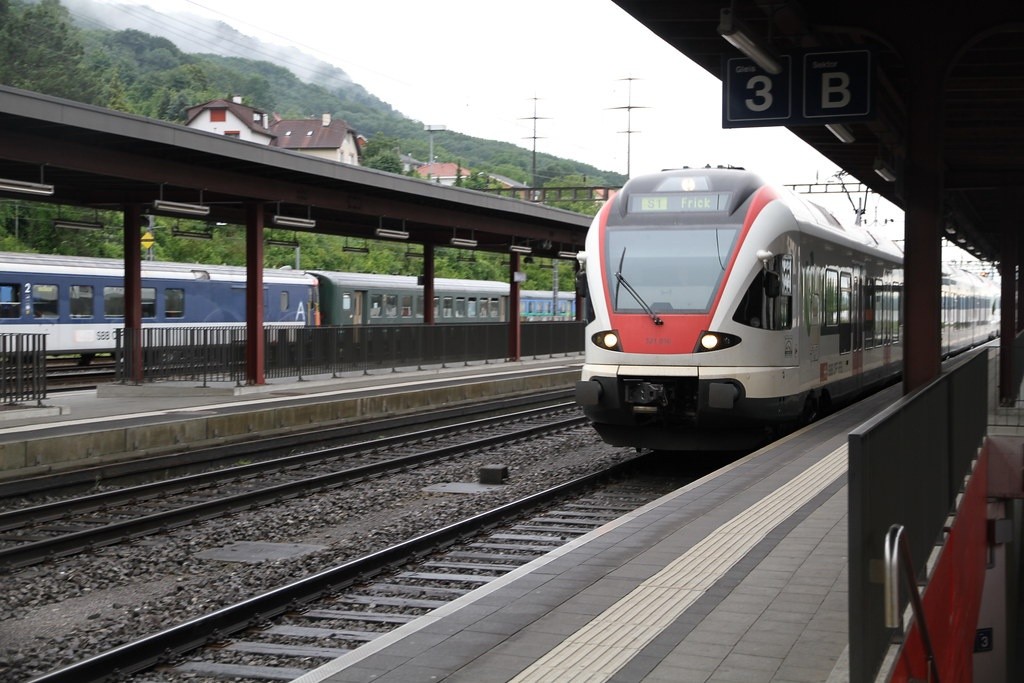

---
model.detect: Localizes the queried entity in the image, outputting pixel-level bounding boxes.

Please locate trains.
[575,164,1001,451]
[0,252,576,362]
